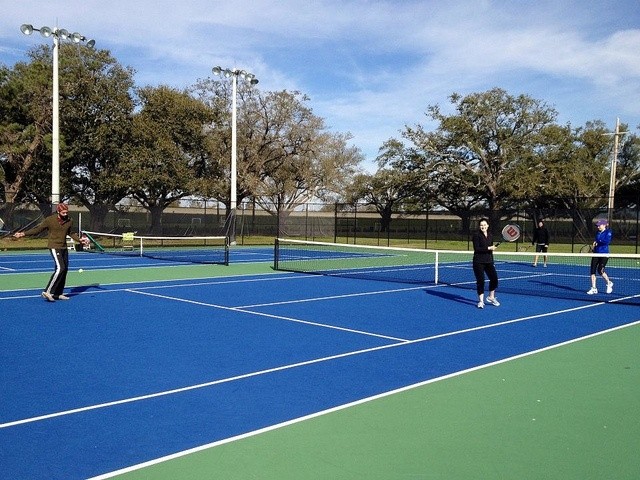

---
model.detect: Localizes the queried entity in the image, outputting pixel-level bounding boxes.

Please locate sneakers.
[606,281,613,294]
[42,292,54,301]
[533,264,537,266]
[544,265,547,267]
[477,301,485,309]
[52,294,70,301]
[486,296,501,307]
[587,287,598,295]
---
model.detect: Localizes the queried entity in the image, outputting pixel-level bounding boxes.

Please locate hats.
[56,204,68,211]
[596,219,608,226]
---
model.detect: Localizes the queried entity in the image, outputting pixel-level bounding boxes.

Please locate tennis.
[637,262,640,265]
[78,268,83,274]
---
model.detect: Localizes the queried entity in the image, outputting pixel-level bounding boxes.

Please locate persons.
[586,220,614,295]
[14,204,88,302]
[532,219,550,268]
[75,233,91,251]
[472,218,501,308]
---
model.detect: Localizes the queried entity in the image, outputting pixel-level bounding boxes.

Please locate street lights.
[211,67,259,246]
[20,25,95,214]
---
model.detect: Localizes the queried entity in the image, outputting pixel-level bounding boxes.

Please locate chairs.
[66,235,90,250]
